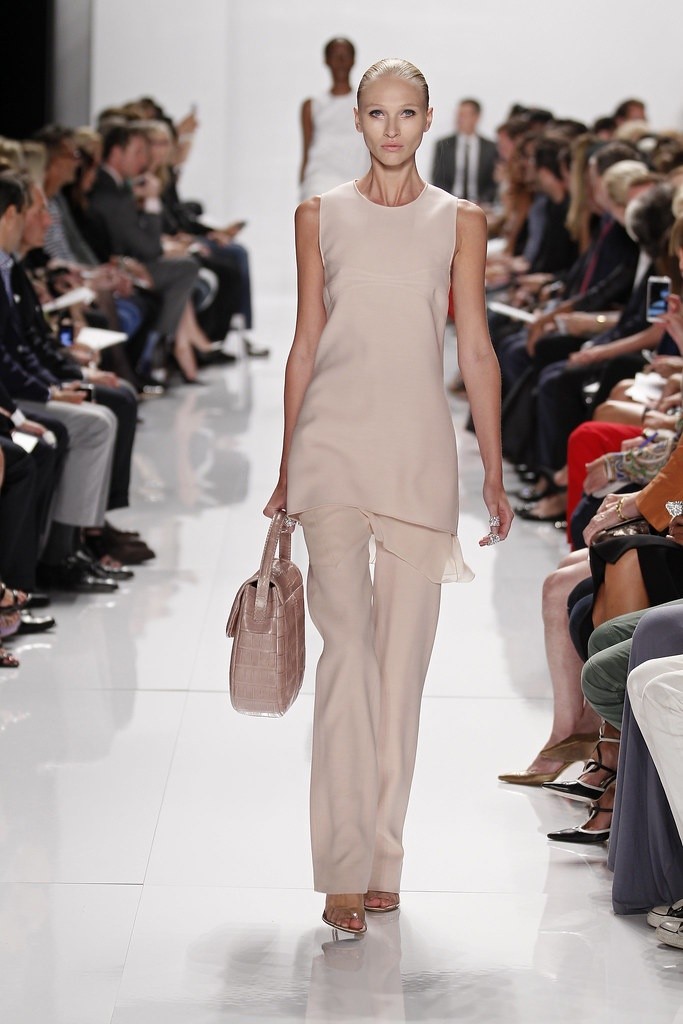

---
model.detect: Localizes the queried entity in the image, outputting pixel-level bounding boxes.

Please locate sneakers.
[647,899,683,948]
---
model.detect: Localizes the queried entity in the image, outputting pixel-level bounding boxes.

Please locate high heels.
[547,806,621,844]
[539,735,621,804]
[511,473,569,529]
[498,760,586,785]
[171,339,224,385]
[540,732,602,760]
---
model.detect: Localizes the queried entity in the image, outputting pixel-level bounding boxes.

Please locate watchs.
[641,426,657,445]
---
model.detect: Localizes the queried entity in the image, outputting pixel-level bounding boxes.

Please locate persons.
[0,97,270,668]
[262,59,514,936]
[449,101,683,953]
[295,38,373,203]
[429,98,494,200]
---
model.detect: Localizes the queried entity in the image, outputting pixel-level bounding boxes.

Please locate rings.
[489,515,500,527]
[280,528,289,534]
[489,534,498,544]
[283,516,295,524]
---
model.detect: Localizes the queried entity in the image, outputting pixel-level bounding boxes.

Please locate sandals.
[363,891,401,913]
[323,891,367,934]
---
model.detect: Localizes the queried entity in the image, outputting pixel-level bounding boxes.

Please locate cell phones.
[75,385,93,402]
[58,325,73,348]
[647,275,671,323]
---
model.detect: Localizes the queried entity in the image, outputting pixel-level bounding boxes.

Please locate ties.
[462,138,469,200]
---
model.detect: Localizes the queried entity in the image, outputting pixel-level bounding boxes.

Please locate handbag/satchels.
[225,509,305,717]
[589,515,652,545]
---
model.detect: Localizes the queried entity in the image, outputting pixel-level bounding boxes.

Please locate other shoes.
[210,352,236,365]
[514,457,542,484]
[58,520,155,592]
[0,582,54,667]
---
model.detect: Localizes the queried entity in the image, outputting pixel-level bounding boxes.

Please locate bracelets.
[596,313,605,332]
[617,496,627,522]
